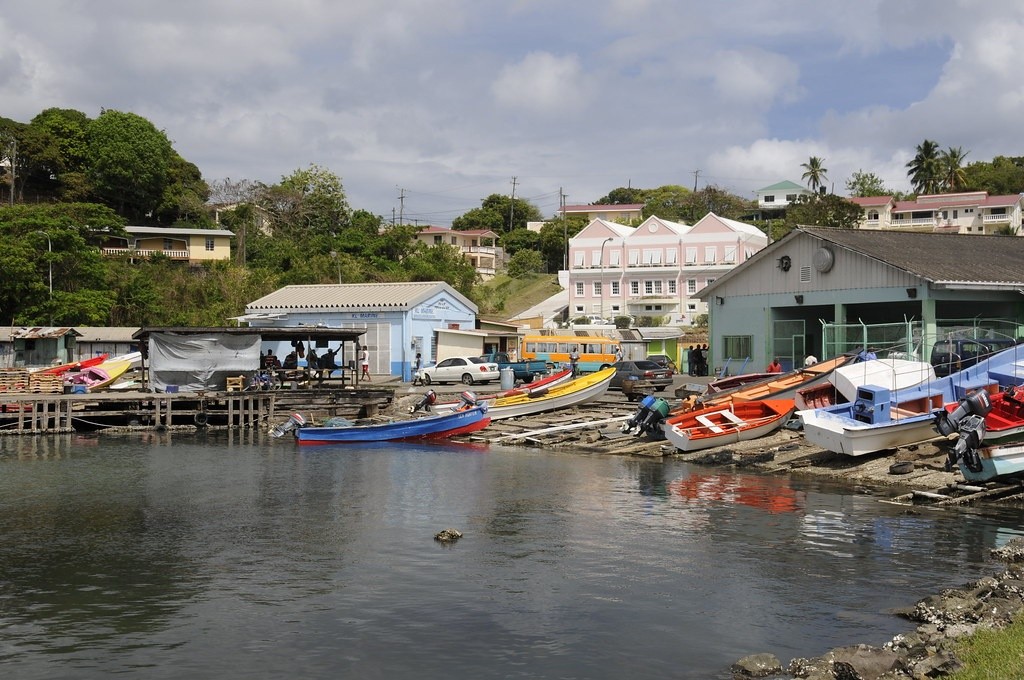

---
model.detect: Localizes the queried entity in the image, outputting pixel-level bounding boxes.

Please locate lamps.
[906,288,917,297]
[794,294,803,304]
[716,296,724,305]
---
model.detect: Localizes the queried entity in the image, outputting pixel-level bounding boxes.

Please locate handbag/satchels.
[415,372,420,379]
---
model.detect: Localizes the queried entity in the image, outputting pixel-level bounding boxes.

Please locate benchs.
[276,367,350,388]
[695,410,747,433]
[955,376,999,400]
[767,381,789,389]
[891,389,944,413]
[731,391,752,403]
[987,413,1011,428]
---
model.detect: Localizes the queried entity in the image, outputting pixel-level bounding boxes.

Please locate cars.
[561,314,636,329]
[646,354,679,376]
[609,360,674,392]
[417,355,501,386]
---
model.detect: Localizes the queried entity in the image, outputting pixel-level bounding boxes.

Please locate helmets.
[805,356,817,367]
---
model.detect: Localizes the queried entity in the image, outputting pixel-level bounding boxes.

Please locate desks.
[227,378,243,391]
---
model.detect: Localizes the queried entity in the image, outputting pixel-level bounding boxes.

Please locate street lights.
[601,237,614,324]
[37,230,53,328]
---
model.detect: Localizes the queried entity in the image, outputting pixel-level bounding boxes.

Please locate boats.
[707,325,1024,487]
[664,398,796,453]
[406,368,573,415]
[0,351,133,413]
[270,400,491,447]
[450,366,617,421]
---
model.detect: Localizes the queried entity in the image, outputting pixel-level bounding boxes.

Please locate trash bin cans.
[501,369,515,390]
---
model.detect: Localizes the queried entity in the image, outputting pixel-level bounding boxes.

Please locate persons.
[321,344,343,378]
[283,351,297,369]
[856,344,877,362]
[306,350,319,377]
[569,348,580,379]
[260,349,281,369]
[805,353,818,366]
[411,353,426,386]
[359,346,372,381]
[613,347,623,363]
[687,344,709,377]
[766,357,781,373]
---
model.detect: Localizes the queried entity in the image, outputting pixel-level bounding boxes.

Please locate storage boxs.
[167,385,178,393]
[73,384,87,394]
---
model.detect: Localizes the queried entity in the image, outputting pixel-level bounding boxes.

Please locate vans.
[888,336,923,361]
[930,338,1024,378]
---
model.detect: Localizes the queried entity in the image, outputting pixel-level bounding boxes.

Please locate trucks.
[478,351,548,384]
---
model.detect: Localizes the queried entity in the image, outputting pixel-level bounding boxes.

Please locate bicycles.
[249,367,282,392]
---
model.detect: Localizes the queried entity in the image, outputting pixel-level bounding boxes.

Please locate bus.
[521,335,622,372]
